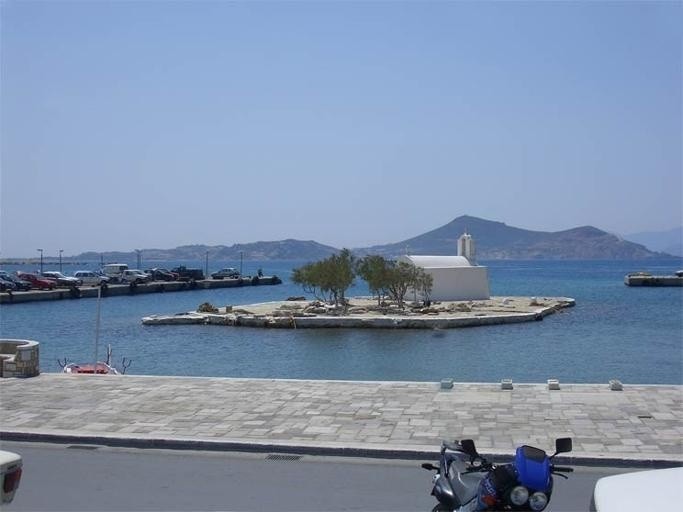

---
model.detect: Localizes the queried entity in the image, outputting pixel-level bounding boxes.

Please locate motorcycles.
[421,436,574,512]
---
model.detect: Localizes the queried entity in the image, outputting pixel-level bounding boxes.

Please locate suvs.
[211,267,241,279]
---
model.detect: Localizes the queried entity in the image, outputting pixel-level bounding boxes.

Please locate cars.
[0,263,206,292]
